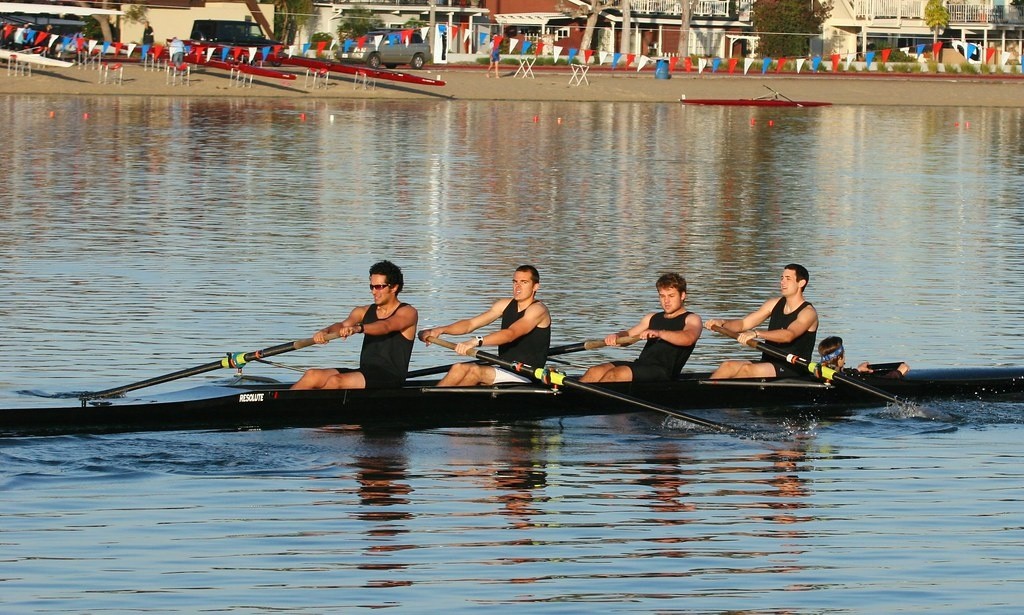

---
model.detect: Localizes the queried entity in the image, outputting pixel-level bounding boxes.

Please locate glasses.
[369,283,394,290]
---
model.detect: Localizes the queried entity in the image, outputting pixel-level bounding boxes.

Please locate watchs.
[356,322,364,333]
[476,335,483,347]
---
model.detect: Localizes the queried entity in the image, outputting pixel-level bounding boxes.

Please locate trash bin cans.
[656,60,671,79]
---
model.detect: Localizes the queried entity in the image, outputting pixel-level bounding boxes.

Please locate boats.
[0,367,1024,436]
[108,42,296,81]
[679,99,833,106]
[0,48,74,67]
[167,40,447,87]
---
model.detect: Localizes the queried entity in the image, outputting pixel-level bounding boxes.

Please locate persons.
[818,335,909,380]
[142,22,154,61]
[23,23,32,40]
[45,24,56,55]
[170,36,189,75]
[578,272,703,382]
[73,29,88,64]
[289,259,418,391]
[485,33,501,78]
[418,264,552,386]
[704,263,819,379]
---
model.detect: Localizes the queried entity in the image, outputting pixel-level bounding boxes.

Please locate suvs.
[189,19,284,67]
[333,29,432,69]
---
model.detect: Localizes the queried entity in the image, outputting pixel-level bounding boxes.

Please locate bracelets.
[753,329,757,338]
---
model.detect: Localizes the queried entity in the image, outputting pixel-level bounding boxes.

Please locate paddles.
[244,359,494,382]
[418,330,741,433]
[87,329,356,399]
[711,324,921,408]
